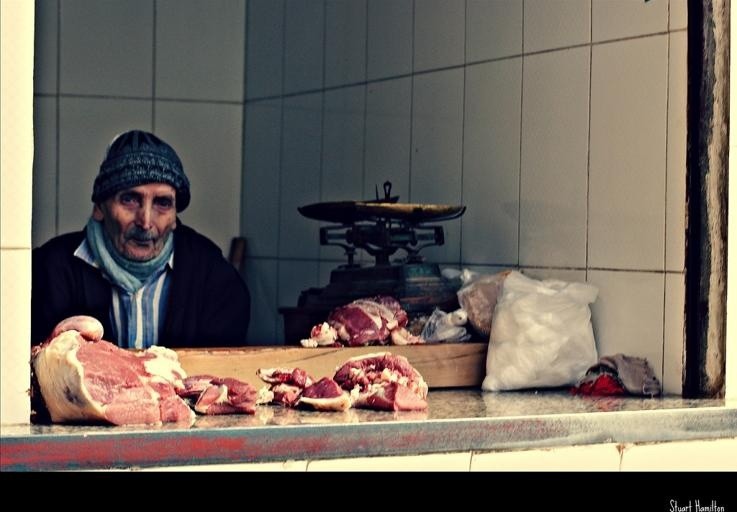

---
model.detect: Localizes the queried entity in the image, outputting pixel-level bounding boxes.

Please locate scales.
[297,201,467,309]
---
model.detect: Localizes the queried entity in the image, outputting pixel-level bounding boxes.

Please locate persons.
[33,127,253,353]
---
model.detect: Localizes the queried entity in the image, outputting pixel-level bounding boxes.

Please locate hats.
[91,130,191,214]
